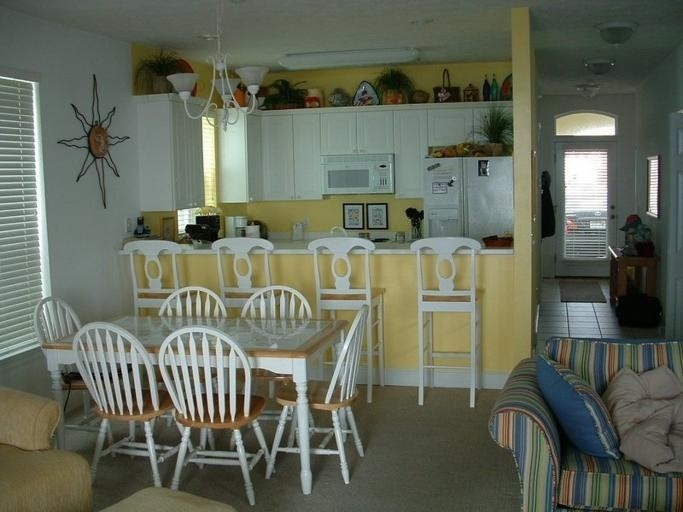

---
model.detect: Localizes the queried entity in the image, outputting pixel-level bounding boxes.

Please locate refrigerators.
[422,157,513,245]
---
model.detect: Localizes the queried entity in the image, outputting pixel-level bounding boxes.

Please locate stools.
[121,239,202,315]
[410,238,484,409]
[98,486,237,512]
[209,238,290,318]
[307,236,387,405]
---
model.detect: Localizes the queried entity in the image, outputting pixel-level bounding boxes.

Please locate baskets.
[432,68,459,103]
[264,81,304,109]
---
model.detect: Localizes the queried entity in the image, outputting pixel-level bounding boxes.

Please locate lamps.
[586,58,609,74]
[576,85,600,96]
[595,20,638,45]
[166,0,270,132]
[278,48,419,71]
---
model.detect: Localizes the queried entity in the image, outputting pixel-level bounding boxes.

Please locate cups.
[245,225,259,238]
[394,231,405,243]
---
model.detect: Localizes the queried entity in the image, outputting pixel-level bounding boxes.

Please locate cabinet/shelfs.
[217,101,513,203]
[132,93,205,212]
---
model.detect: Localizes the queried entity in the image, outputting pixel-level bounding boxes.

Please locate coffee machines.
[195,215,220,233]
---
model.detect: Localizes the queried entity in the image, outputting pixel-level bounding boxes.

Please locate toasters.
[184,223,217,242]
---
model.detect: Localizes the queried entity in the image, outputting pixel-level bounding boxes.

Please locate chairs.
[155,327,267,506]
[233,284,313,448]
[70,325,165,488]
[276,309,369,486]
[34,299,92,433]
[150,285,232,449]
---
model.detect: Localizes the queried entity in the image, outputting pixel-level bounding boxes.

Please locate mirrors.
[646,155,660,219]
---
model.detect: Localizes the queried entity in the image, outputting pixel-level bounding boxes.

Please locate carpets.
[559,281,607,303]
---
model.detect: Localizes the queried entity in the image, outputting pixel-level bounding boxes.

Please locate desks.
[609,247,660,306]
[94,316,348,495]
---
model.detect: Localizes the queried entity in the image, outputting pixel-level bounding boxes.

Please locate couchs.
[0,386,93,512]
[487,336,683,512]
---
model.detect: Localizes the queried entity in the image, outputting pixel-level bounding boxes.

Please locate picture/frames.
[343,203,364,230]
[366,203,388,230]
[160,216,175,242]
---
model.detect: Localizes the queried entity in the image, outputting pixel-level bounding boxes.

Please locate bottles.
[482,73,489,102]
[302,218,308,240]
[224,216,246,237]
[489,72,499,101]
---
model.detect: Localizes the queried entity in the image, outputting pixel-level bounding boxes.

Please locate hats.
[618,214,640,231]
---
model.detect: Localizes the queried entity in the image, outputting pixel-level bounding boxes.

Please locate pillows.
[536,354,622,460]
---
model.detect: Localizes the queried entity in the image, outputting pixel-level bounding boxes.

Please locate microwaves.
[320,153,393,195]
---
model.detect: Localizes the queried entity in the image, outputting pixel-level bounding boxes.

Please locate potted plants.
[135,48,185,94]
[373,66,417,105]
[259,79,308,109]
